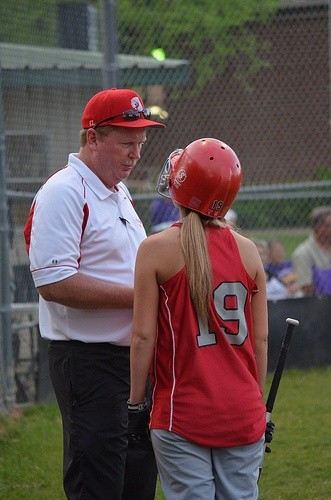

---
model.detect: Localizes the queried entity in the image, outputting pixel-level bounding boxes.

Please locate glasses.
[93,108,152,129]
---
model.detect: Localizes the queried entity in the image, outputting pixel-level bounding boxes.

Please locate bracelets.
[127,396,149,413]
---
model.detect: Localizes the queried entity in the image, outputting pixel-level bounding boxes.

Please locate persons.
[245,235,304,300]
[290,206,331,296]
[22,86,168,500]
[127,137,270,500]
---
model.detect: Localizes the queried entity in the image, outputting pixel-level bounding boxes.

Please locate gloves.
[126,397,151,449]
[265,422,275,453]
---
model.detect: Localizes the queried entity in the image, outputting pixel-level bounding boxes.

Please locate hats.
[80,87,167,129]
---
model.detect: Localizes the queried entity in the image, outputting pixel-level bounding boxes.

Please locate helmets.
[164,137,243,220]
